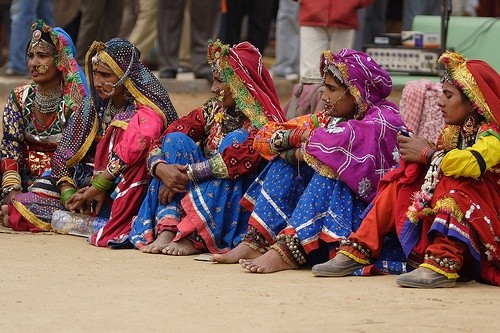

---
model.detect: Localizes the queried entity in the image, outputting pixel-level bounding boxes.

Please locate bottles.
[51,210,109,238]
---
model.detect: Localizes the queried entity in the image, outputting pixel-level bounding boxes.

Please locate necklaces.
[101,98,125,137]
[224,109,246,129]
[35,84,64,112]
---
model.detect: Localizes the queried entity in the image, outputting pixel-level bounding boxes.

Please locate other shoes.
[395,267,457,288]
[195,70,213,85]
[159,68,177,79]
[271,70,299,83]
[312,258,365,276]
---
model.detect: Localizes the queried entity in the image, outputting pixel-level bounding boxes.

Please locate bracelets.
[285,147,298,166]
[149,159,168,179]
[420,147,438,164]
[91,172,113,191]
[0,169,24,197]
[184,163,194,183]
[61,188,78,205]
[289,126,306,148]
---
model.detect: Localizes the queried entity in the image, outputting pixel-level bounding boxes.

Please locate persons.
[1,1,305,80]
[210,48,414,274]
[310,50,500,289]
[52,38,180,248]
[0,19,86,232]
[128,42,288,256]
[292,0,500,85]
